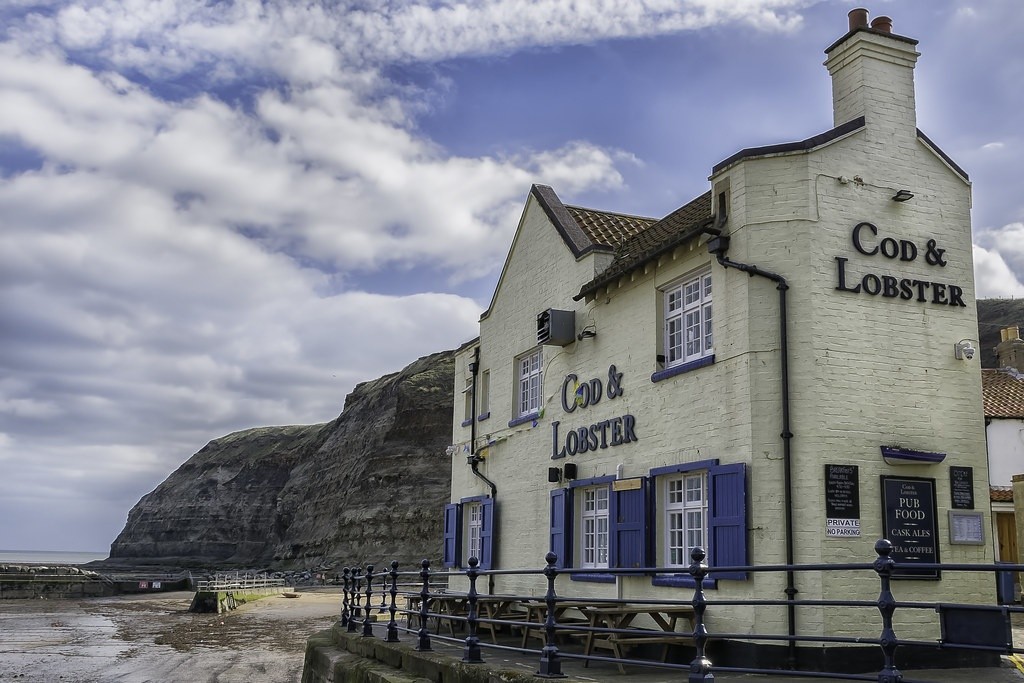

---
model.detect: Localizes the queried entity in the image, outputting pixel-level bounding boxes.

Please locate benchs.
[399,610,708,649]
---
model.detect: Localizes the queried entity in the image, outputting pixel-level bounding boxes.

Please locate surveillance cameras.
[963,348,975,359]
[446,449,453,455]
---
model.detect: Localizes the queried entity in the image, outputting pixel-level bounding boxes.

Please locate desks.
[400,592,709,676]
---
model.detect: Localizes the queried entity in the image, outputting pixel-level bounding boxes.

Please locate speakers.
[564,463,576,479]
[548,467,558,482]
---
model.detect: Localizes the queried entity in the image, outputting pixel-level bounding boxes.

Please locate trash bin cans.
[995,561,1019,606]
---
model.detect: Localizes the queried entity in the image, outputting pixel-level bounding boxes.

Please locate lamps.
[953,338,980,360]
[891,189,915,202]
